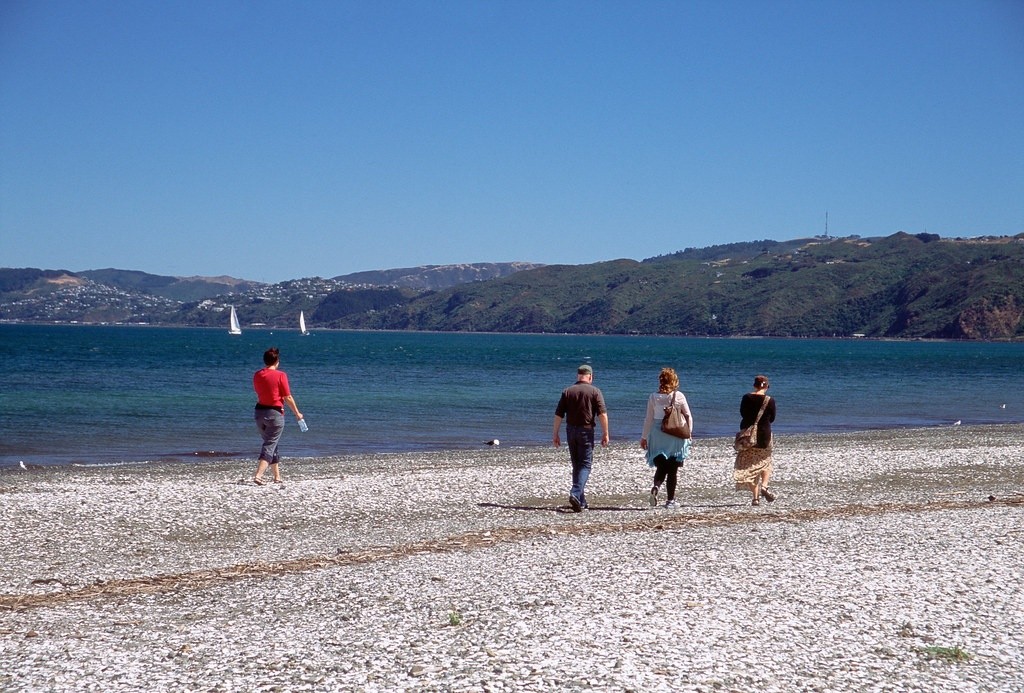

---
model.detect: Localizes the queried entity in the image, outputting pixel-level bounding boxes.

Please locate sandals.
[761,486,775,503]
[752,498,760,506]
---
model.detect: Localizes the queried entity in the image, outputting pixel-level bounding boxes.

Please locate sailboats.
[299,310,310,336]
[228,305,241,334]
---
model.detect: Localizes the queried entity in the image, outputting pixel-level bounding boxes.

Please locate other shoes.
[254,477,266,486]
[274,478,282,482]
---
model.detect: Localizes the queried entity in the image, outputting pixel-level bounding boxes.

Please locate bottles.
[298,418,308,432]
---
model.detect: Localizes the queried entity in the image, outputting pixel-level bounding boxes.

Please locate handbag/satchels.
[662,390,691,439]
[734,425,758,452]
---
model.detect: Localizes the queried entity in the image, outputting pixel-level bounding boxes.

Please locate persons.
[641,367,694,510]
[552,363,611,514]
[733,373,776,509]
[251,347,305,486]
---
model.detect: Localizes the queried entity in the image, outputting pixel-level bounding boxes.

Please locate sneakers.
[650,486,659,507]
[570,496,589,514]
[666,500,682,509]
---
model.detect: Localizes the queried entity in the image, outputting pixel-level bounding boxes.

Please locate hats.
[577,364,597,374]
